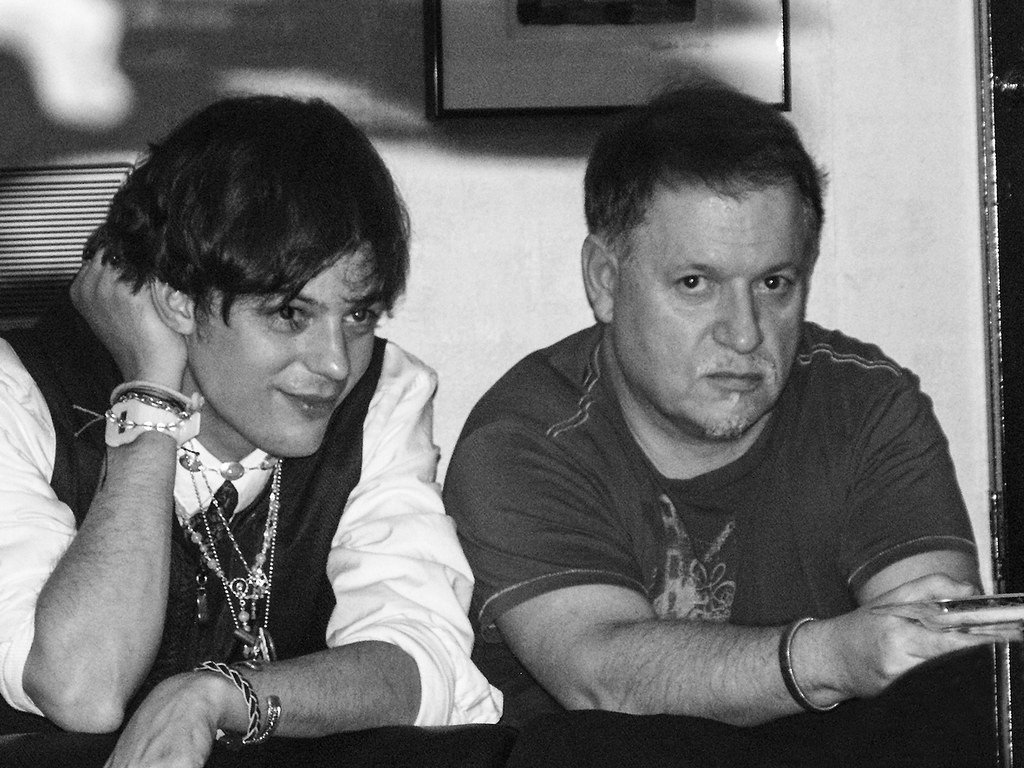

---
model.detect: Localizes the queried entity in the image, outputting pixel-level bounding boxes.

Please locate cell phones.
[929,593,1024,627]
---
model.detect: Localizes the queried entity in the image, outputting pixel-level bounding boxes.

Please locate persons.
[0,91,502,768]
[443,71,1006,726]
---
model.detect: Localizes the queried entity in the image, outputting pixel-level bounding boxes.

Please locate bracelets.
[779,612,839,714]
[193,660,283,749]
[69,379,206,448]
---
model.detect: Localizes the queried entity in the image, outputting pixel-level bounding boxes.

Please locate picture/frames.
[422,0,793,122]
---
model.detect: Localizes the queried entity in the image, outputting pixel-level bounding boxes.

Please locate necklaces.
[172,431,284,667]
